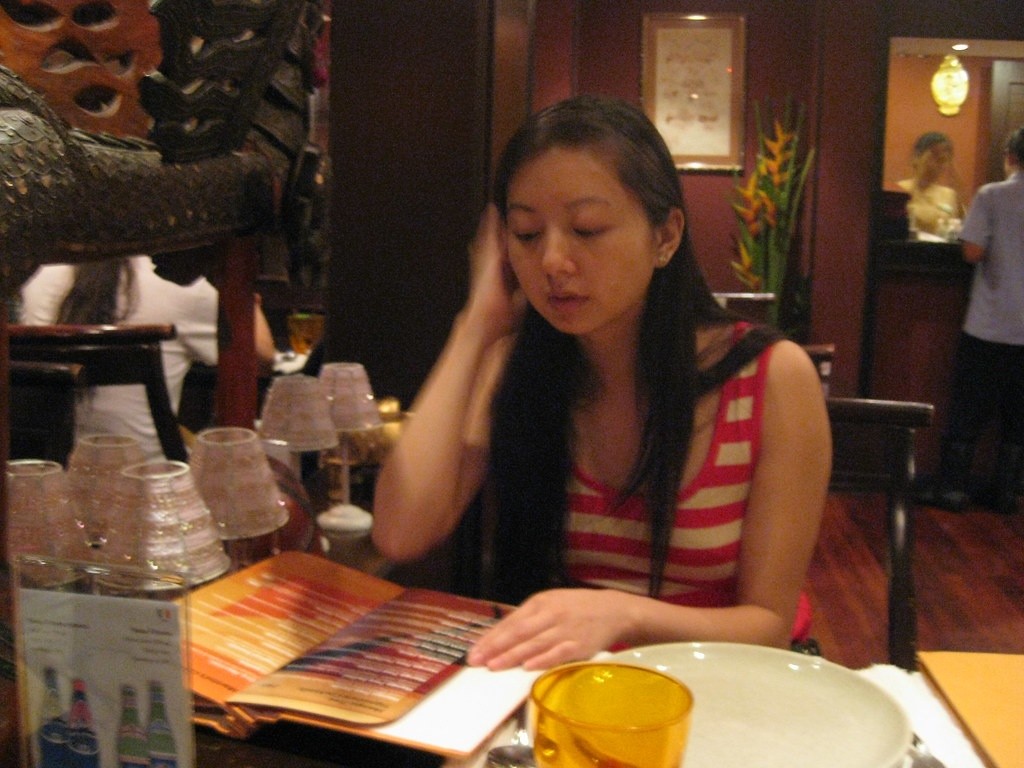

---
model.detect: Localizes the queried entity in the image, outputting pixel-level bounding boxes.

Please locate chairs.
[822,394,940,677]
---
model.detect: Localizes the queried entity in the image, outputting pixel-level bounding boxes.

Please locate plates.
[609,638,913,768]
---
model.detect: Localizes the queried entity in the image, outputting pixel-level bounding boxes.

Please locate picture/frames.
[639,11,748,177]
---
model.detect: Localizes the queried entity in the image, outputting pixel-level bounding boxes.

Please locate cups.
[527,664,694,767]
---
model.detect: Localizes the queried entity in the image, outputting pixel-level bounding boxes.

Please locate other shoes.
[915,480,980,504]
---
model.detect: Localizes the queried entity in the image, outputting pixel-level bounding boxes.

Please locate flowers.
[724,94,816,329]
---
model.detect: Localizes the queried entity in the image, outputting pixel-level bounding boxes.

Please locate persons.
[373,96,834,672]
[901,131,971,238]
[18,254,277,464]
[915,129,1024,510]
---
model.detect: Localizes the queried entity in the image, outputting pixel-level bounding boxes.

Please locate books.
[170,551,549,758]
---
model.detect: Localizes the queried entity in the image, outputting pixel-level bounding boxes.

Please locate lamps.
[930,55,969,118]
[3,362,382,596]
